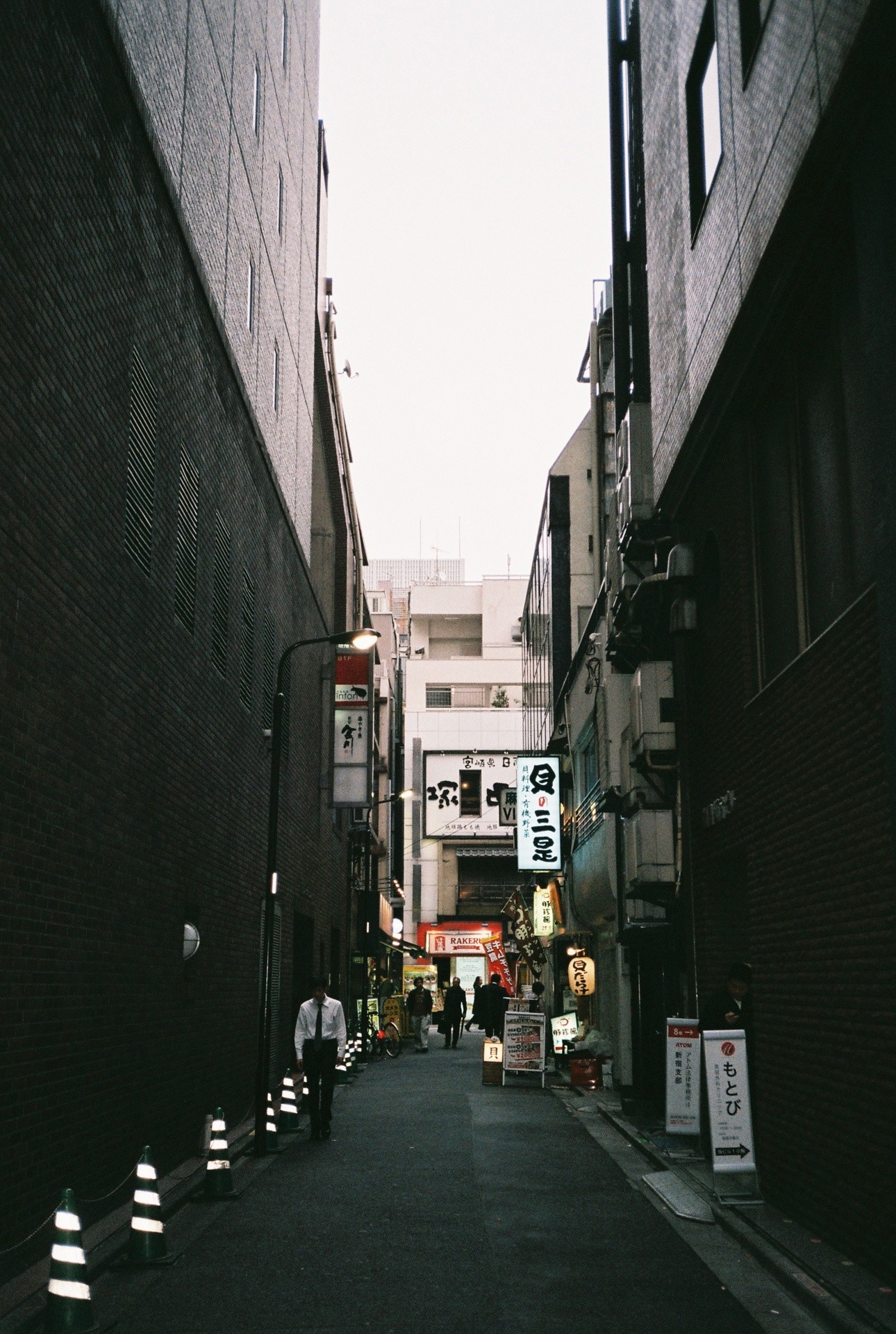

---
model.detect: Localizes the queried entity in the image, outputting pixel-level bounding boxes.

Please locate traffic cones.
[277,1067,302,1133]
[41,1188,101,1334]
[334,1030,362,1084]
[120,1144,183,1266]
[202,1106,238,1199]
[264,1091,286,1153]
[299,1071,310,1114]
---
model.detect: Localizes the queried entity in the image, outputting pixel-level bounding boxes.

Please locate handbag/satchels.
[437,1019,446,1035]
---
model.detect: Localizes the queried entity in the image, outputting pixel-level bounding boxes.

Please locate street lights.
[253,627,380,1153]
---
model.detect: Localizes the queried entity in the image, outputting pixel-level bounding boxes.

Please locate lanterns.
[568,956,595,996]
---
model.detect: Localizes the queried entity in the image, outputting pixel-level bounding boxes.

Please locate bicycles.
[349,1001,402,1059]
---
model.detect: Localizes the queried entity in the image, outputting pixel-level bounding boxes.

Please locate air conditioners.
[632,660,676,753]
[623,807,674,897]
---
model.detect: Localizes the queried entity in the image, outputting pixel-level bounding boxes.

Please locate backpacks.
[528,996,543,1012]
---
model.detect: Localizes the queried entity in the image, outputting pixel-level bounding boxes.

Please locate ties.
[314,1003,323,1052]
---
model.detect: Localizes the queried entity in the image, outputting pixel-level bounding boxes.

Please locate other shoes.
[452,1042,456,1048]
[322,1123,331,1139]
[465,1025,472,1032]
[442,1042,450,1048]
[422,1048,429,1053]
[415,1049,422,1053]
[309,1130,320,1142]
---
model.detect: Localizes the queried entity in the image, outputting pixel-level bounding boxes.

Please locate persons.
[475,974,509,1043]
[464,977,486,1032]
[405,977,433,1052]
[379,968,393,1030]
[295,976,347,1139]
[441,977,467,1048]
[698,960,755,1165]
[520,982,546,1014]
